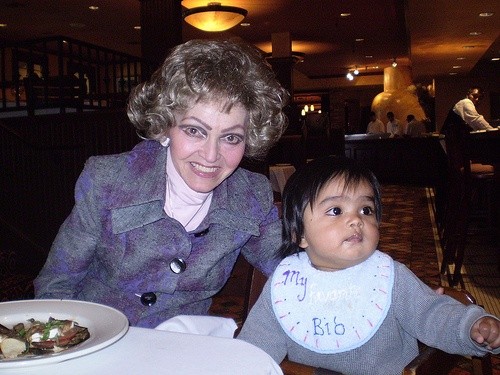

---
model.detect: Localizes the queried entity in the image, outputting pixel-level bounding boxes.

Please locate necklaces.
[166,175,213,226]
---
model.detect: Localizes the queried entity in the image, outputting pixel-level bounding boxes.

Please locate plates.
[0,299,128,369]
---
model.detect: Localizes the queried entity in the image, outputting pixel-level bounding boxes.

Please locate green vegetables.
[18,318,66,341]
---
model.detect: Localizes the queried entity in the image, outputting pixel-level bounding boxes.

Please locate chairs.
[410,283,493,375]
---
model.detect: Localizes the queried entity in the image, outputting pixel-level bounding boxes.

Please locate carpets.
[242,179,442,335]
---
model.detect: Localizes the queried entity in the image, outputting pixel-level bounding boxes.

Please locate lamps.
[181,0,248,33]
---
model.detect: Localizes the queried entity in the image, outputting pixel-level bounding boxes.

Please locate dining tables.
[269,164,297,202]
[0,327,286,375]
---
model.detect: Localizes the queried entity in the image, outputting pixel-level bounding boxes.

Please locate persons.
[366,111,385,134]
[386,111,403,135]
[440,86,493,158]
[36,40,283,330]
[233,158,500,375]
[406,114,426,136]
